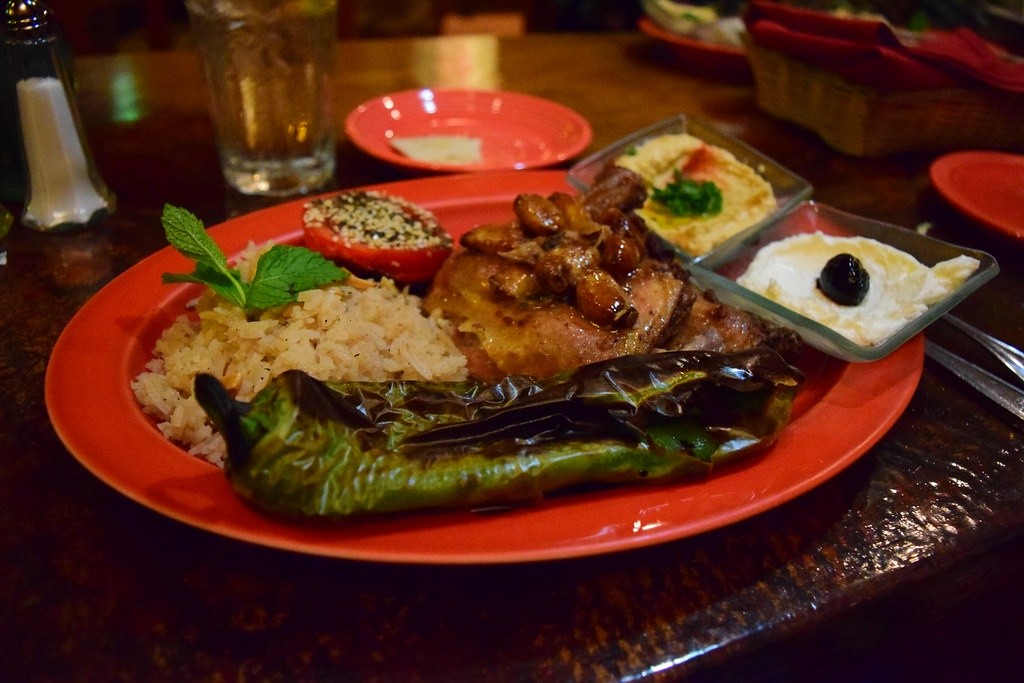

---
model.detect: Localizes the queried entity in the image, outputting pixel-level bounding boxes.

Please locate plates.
[929,150,1024,244]
[687,199,1001,365]
[45,171,926,565]
[567,111,814,269]
[344,87,594,177]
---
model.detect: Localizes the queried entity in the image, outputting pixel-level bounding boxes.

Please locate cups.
[186,0,339,197]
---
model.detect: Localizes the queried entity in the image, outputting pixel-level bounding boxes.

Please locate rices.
[131,239,474,469]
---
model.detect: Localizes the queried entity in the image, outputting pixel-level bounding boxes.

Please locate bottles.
[3,0,117,235]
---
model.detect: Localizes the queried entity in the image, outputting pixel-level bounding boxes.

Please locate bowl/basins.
[636,17,748,75]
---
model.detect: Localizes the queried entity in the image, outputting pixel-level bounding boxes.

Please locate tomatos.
[300,195,456,287]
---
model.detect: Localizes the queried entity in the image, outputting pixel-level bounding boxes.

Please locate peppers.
[195,347,806,518]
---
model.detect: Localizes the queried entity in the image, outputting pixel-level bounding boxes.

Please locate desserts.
[737,231,981,347]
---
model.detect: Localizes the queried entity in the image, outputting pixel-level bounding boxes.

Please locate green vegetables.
[642,168,723,216]
[160,204,349,316]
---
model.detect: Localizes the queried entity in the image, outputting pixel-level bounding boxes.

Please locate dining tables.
[0,31,1024,683]
